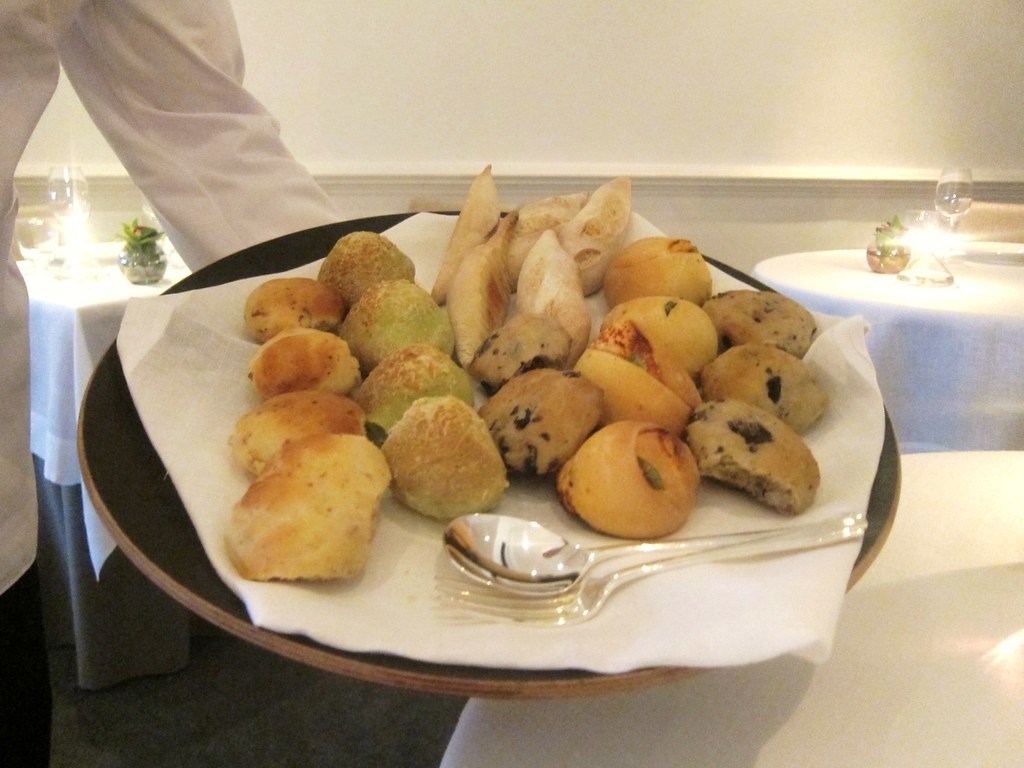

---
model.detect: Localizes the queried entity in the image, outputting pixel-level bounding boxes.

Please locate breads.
[218,166,826,580]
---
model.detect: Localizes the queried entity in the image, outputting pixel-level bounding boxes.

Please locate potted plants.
[865,215,911,275]
[118,218,171,286]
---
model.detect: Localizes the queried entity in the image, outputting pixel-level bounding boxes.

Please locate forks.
[438,523,868,624]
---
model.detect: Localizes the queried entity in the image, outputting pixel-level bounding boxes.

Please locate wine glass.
[47,166,92,268]
[17,220,55,297]
[934,167,973,259]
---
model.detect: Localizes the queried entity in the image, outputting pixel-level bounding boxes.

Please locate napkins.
[114,200,885,673]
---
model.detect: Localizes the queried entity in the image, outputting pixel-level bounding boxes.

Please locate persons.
[0,0,354,768]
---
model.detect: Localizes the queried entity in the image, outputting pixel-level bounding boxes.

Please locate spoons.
[443,514,867,597]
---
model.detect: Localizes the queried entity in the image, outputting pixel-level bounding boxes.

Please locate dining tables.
[438,453,1024,766]
[748,249,1024,451]
[12,236,193,692]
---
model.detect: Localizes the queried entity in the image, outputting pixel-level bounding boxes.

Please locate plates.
[76,211,902,699]
